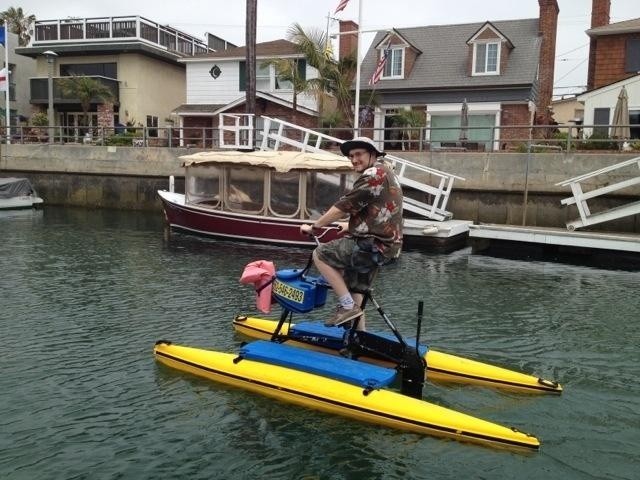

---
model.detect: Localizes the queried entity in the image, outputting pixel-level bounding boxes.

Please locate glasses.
[348,151,369,159]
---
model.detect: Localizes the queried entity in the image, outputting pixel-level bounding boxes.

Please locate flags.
[334,0,349,14]
[368,38,392,86]
[0,27,5,48]
[0,68,7,91]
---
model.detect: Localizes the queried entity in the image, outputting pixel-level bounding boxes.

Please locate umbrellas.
[610,85,631,149]
[458,98,470,146]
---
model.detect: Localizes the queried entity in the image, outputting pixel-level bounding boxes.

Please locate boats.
[156,148,357,249]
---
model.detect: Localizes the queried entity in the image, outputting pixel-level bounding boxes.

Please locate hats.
[340,136,387,156]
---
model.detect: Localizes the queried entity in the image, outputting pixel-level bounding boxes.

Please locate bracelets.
[299,136,403,357]
[312,223,321,232]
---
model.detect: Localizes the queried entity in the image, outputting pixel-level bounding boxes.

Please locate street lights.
[42,49,58,142]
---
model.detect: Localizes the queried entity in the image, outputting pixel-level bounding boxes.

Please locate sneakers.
[325,303,364,327]
